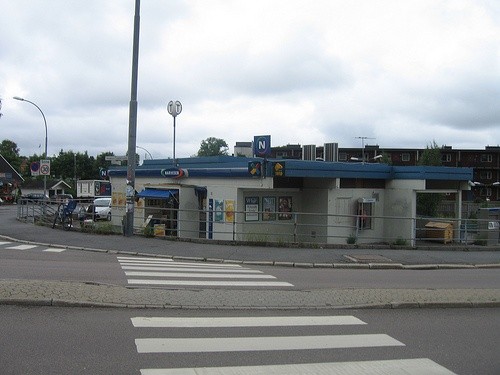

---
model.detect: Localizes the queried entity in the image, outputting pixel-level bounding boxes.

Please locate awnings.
[136,189,179,199]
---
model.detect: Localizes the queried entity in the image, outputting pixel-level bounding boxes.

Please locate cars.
[85,197,112,221]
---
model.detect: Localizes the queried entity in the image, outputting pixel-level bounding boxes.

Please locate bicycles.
[51,202,72,231]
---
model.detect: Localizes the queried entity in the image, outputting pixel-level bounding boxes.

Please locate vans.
[30,192,48,199]
[49,193,74,204]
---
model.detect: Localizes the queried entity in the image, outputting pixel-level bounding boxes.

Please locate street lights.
[12,96,48,216]
